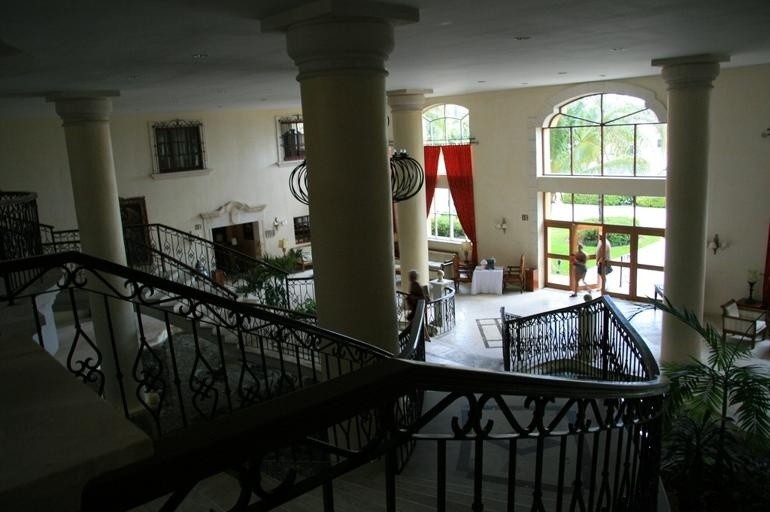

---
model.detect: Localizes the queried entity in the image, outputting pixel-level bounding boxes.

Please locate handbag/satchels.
[598,262,612,276]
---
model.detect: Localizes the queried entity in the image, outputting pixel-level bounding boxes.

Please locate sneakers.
[569,294,577,297]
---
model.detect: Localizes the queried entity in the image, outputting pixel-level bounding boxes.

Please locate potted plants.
[748,270,757,299]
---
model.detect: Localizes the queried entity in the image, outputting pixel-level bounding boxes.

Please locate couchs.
[428,251,459,288]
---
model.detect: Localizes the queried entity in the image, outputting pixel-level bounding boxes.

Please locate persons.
[594,232,612,293]
[403,268,428,327]
[569,243,591,297]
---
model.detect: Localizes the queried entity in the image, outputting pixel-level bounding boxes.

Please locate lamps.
[708,234,728,257]
[495,218,508,235]
[273,218,282,232]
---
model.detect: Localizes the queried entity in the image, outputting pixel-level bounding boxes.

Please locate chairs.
[721,298,766,349]
[453,254,525,294]
